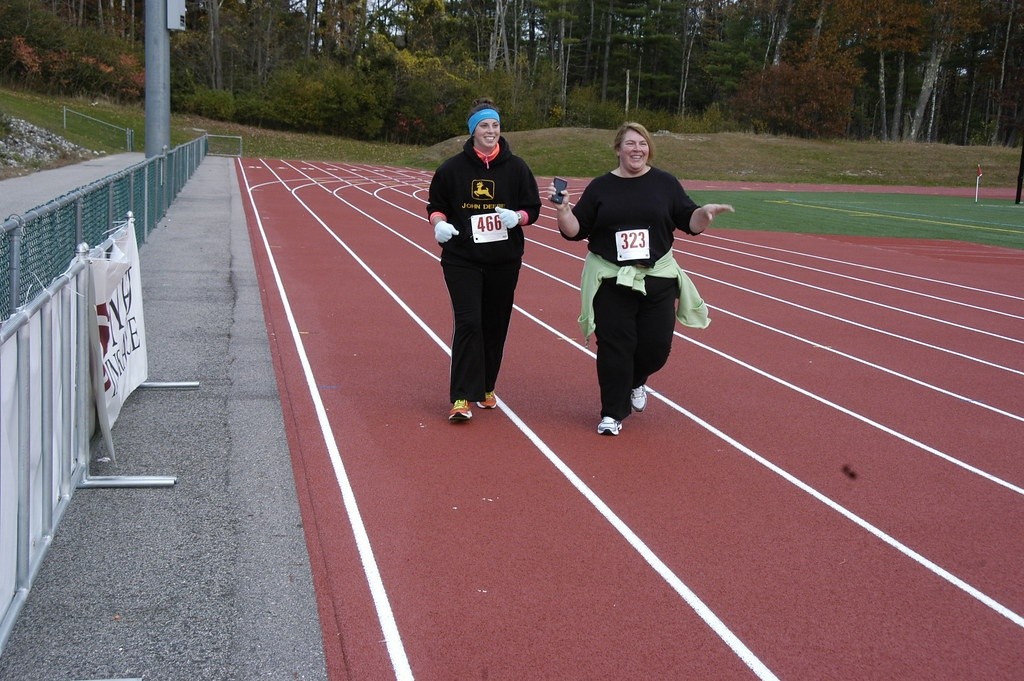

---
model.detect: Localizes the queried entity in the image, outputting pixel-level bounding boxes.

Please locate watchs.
[514,211,522,225]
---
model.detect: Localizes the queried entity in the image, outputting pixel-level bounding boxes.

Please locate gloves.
[433,221,459,244]
[494,206,518,229]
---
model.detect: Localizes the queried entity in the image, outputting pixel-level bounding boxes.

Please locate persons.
[426,103,542,423]
[547,122,735,436]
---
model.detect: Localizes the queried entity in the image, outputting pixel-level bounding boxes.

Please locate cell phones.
[550,176,567,204]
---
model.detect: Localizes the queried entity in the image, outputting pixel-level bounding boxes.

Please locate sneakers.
[475,391,497,409]
[596,416,622,436]
[631,385,648,412]
[448,400,472,421]
